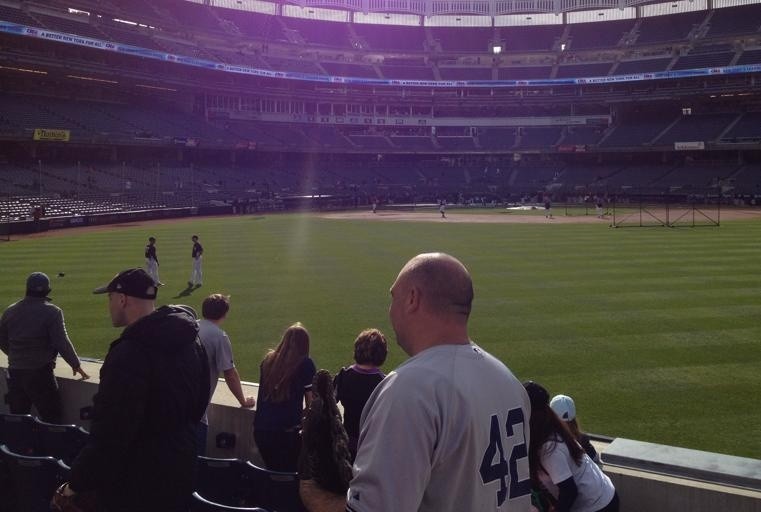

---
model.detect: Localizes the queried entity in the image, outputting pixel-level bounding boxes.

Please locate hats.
[93,269,157,299]
[549,395,576,422]
[26,272,50,295]
[523,382,547,406]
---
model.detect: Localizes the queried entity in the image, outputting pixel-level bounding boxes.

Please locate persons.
[28,202,52,233]
[550,394,605,470]
[189,235,204,287]
[145,237,165,286]
[126,154,761,220]
[50,266,211,512]
[0,272,89,425]
[334,328,388,465]
[254,326,316,470]
[187,294,256,457]
[296,251,534,511]
[343,106,435,136]
[523,382,620,512]
